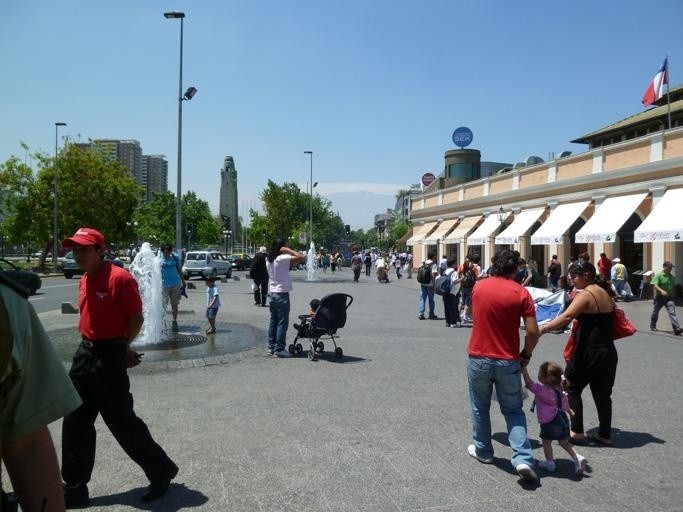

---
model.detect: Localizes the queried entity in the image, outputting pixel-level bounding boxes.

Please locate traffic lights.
[221,215,231,230]
[186,223,193,237]
[344,225,350,236]
[262,228,266,238]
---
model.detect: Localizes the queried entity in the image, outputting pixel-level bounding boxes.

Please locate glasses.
[160,246,171,252]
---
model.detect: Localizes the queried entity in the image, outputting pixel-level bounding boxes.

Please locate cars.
[227,255,255,271]
[63,248,124,278]
[33,250,54,257]
[0,257,42,294]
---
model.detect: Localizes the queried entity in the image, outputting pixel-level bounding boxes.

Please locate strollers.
[288,292,353,362]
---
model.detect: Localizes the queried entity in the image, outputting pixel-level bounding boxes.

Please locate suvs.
[181,250,233,281]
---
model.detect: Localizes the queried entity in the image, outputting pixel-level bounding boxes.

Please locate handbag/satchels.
[613,303,637,340]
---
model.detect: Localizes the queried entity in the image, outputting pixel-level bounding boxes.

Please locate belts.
[78,338,106,348]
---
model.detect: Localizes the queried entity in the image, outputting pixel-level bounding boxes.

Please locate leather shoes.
[143,478,170,502]
[62,484,88,504]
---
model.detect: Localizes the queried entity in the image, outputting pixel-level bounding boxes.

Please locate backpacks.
[417,260,434,284]
[435,271,454,296]
[460,265,476,289]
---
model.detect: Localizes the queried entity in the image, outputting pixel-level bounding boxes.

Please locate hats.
[61,226,107,250]
[180,284,188,298]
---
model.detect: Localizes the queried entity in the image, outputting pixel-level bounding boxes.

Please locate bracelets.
[521,350,532,360]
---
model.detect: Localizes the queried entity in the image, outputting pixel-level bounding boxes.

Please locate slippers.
[569,433,589,446]
[588,432,613,447]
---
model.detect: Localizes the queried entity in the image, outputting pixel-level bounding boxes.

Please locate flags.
[641,56,667,106]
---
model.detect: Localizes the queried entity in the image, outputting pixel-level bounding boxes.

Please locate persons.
[650,261,683,336]
[521,361,586,475]
[321,252,412,282]
[0,258,84,512]
[160,244,186,331]
[418,252,495,328]
[467,249,539,483]
[250,246,270,306]
[567,253,590,293]
[512,251,539,288]
[597,253,628,296]
[539,260,619,445]
[61,227,180,507]
[309,299,320,316]
[548,254,561,293]
[205,276,221,334]
[265,238,304,358]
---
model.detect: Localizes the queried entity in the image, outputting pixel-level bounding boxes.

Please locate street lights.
[304,151,319,282]
[165,10,196,271]
[52,121,67,273]
[222,230,232,257]
[376,220,386,256]
[125,221,139,262]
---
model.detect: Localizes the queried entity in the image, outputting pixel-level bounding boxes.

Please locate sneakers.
[538,459,557,473]
[419,315,461,328]
[467,442,495,465]
[172,319,177,329]
[206,327,216,334]
[516,461,538,482]
[573,454,587,476]
[267,349,274,356]
[273,350,293,358]
